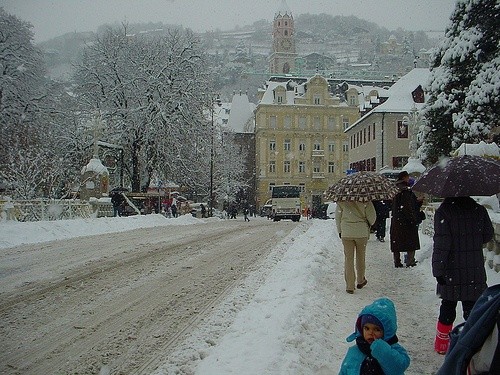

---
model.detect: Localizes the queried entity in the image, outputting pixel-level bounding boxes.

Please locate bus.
[271,186,301,222]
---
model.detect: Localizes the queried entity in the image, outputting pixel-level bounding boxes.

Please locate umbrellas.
[411,154,500,197]
[323,173,402,203]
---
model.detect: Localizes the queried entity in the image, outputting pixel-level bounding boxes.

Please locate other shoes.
[407,264,416,267]
[357,280,367,289]
[378,236,384,242]
[395,264,403,267]
[346,290,354,294]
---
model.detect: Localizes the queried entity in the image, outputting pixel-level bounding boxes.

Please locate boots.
[435,321,452,354]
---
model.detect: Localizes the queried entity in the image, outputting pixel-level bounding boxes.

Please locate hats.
[395,171,415,188]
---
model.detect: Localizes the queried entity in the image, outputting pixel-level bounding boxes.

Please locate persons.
[307,205,327,220]
[431,194,495,356]
[337,296,411,375]
[368,168,427,268]
[111,188,263,222]
[335,201,377,294]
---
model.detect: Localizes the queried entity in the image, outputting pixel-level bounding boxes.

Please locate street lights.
[210,92,222,215]
